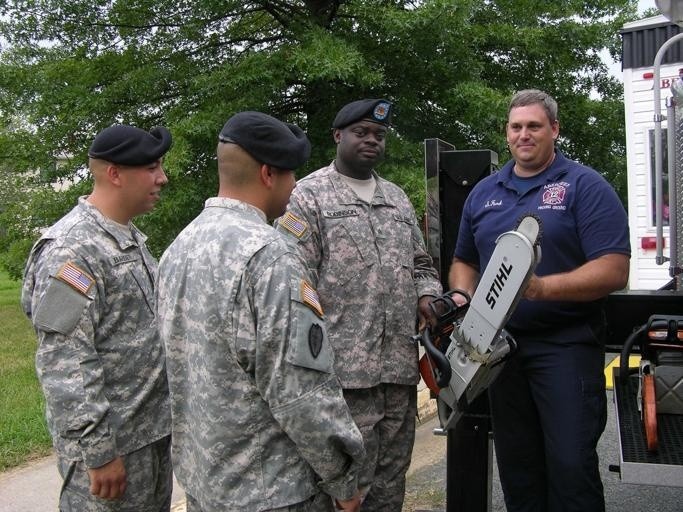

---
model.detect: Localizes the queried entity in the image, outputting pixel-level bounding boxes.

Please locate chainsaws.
[409,211,544,440]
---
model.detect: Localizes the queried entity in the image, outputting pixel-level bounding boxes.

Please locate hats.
[218,110,311,169]
[333,98,392,129]
[88,126,172,167]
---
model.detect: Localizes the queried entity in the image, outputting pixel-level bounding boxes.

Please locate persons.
[153,110,366,512]
[18,124,175,512]
[448,89,632,510]
[271,97,442,511]
